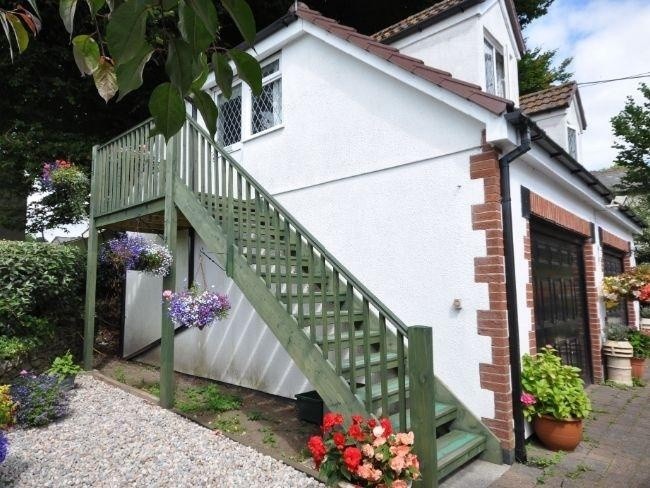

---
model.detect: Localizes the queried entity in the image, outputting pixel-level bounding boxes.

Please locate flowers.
[520,342,595,422]
[39,156,88,189]
[0,382,21,430]
[307,409,424,488]
[10,367,72,432]
[97,233,175,279]
[158,276,233,330]
[601,262,650,310]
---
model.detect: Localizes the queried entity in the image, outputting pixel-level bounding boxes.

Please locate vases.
[533,412,585,451]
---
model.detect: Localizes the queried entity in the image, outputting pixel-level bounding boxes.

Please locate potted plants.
[608,319,649,381]
[44,347,84,392]
